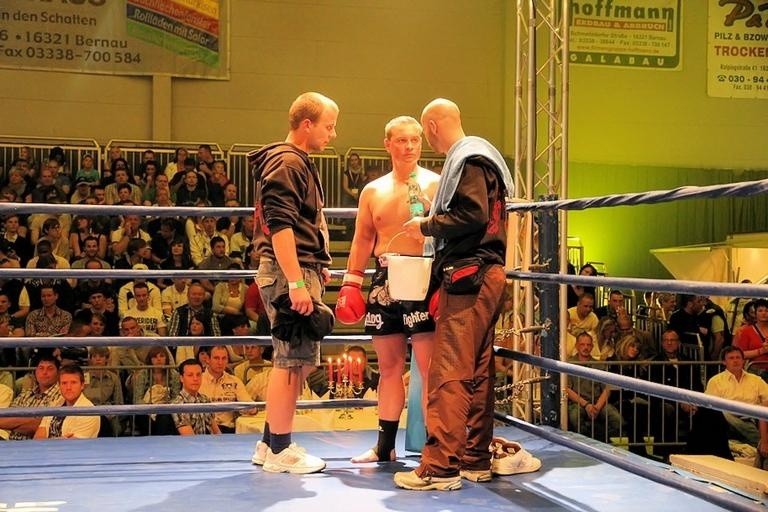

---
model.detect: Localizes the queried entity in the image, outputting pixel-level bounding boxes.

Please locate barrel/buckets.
[380,231,436,302]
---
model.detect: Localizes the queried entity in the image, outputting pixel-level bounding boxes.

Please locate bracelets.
[285,279,306,290]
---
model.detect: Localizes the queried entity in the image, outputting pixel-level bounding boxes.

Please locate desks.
[232,406,408,433]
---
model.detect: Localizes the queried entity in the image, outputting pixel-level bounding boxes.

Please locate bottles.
[407,170,426,223]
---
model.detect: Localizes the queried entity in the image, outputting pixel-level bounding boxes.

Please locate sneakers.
[394,469,462,491]
[252,440,326,474]
[489,436,542,475]
[458,468,491,482]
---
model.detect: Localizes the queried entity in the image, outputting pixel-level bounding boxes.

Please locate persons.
[327,344,378,400]
[333,113,443,464]
[564,254,768,441]
[700,343,768,470]
[342,153,364,208]
[2,144,269,434]
[245,90,340,474]
[387,95,493,491]
[358,165,380,198]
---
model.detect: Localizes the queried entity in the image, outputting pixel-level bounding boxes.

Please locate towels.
[302,388,376,401]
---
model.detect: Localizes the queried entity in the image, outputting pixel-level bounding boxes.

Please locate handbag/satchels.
[441,256,485,294]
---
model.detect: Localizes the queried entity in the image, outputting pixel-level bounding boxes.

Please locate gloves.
[335,271,366,325]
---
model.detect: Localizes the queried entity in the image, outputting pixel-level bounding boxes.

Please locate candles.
[325,353,361,384]
